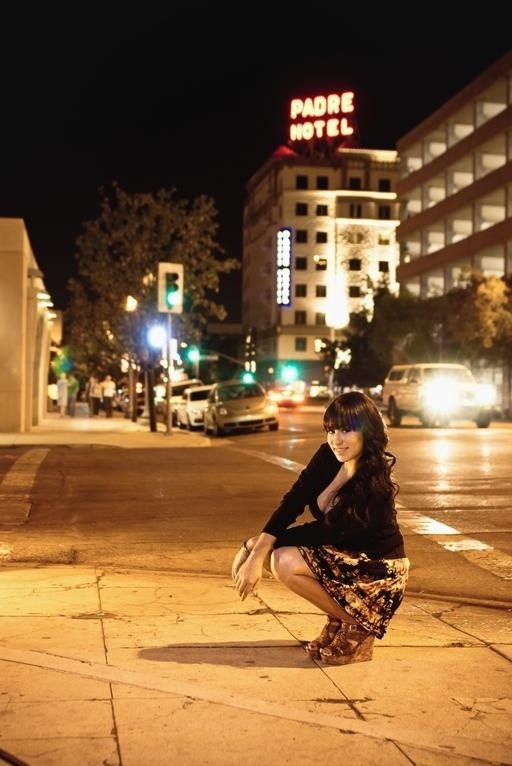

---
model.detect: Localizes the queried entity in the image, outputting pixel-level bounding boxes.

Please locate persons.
[57,373,117,418]
[231,392,411,665]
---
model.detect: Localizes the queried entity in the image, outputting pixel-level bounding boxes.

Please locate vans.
[382,363,496,428]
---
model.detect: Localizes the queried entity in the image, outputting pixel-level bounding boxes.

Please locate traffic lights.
[164,270,180,309]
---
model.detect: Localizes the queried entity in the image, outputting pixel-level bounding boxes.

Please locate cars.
[162,376,204,423]
[176,383,216,430]
[204,378,277,436]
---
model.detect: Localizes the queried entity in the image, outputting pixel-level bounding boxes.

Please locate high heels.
[306,613,375,665]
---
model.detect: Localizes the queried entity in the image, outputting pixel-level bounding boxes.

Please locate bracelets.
[241,542,251,558]
